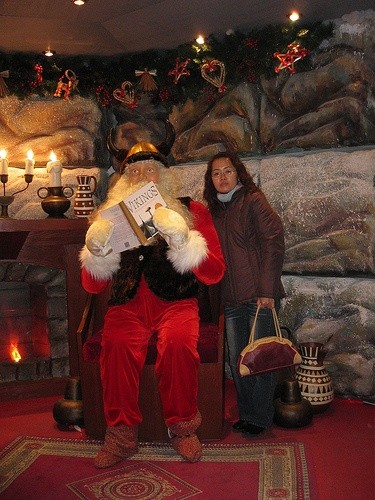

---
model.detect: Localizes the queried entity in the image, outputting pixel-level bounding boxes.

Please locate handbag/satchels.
[237,302,303,376]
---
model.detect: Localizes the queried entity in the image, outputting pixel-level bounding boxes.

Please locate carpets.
[0,434,311,500]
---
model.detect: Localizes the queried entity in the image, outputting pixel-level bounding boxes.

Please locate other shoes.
[232,419,264,438]
[94,441,139,468]
[168,431,203,463]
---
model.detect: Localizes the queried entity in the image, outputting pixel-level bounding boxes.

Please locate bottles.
[294,342,334,415]
[274,379,312,427]
[53,378,84,428]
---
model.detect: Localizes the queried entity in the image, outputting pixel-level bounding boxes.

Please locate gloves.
[84,219,114,258]
[152,207,190,252]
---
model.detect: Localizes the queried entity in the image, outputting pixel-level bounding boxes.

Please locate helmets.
[119,140,169,177]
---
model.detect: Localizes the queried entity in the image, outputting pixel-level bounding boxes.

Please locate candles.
[24,149,35,175]
[46,151,62,187]
[0,149,8,175]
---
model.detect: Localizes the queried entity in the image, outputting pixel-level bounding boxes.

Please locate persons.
[203,152,285,438]
[79,142,225,469]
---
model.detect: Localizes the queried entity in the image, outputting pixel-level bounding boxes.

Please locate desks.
[0,218,111,431]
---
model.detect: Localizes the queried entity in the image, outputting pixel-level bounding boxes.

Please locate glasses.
[211,168,238,179]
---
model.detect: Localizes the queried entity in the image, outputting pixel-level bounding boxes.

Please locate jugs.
[74,175,96,218]
